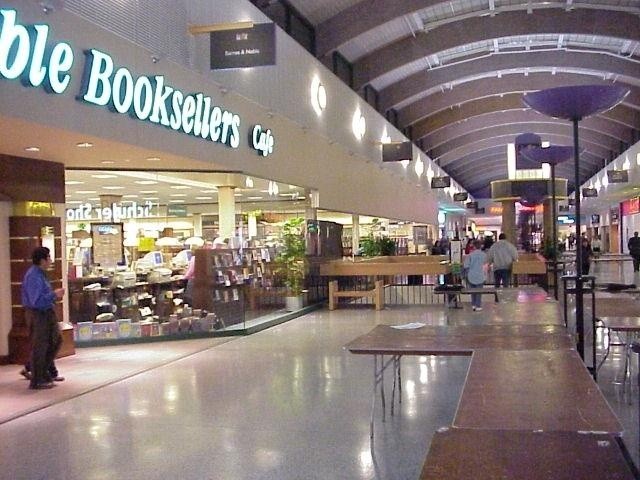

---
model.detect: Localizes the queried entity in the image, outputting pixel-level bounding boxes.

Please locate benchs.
[328,279,384,311]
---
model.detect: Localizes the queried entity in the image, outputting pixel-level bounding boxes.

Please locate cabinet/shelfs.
[191,245,286,330]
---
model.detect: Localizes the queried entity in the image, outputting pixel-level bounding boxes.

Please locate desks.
[348,288,640,479]
[563,252,635,285]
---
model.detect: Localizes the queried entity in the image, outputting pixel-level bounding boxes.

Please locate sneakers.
[29,382,52,389]
[20,369,31,379]
[475,308,483,311]
[50,376,64,381]
[472,306,476,311]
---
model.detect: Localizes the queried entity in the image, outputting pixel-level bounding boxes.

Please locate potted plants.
[274,217,318,312]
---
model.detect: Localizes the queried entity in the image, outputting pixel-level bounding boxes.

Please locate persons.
[432,232,601,311]
[21,247,65,389]
[628,232,640,272]
[183,246,198,279]
[20,309,65,380]
